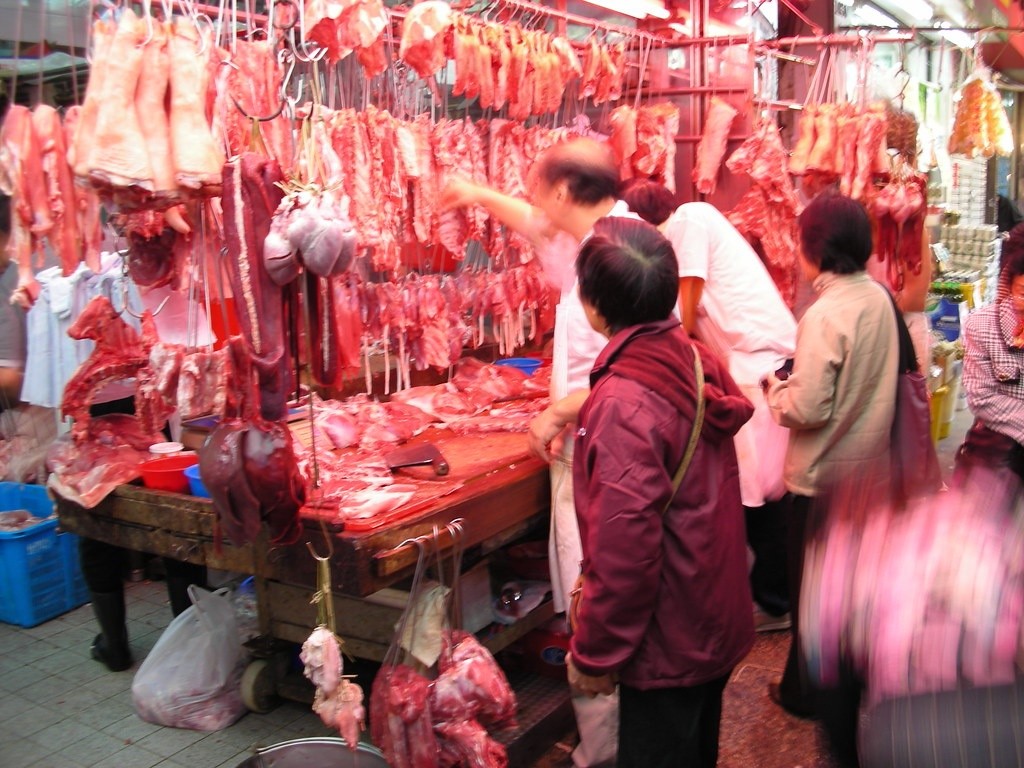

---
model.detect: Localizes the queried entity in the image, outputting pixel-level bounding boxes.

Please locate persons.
[440,143,898,768]
[0,187,115,412]
[963,256,1024,444]
[78,243,218,673]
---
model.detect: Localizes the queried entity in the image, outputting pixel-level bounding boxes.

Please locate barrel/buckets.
[235,736,393,768]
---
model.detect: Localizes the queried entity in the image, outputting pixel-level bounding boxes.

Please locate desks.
[51,363,555,768]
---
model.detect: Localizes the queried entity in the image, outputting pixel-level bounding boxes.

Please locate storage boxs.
[0,480,90,628]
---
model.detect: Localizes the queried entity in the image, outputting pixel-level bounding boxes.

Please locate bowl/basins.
[184,464,213,499]
[497,358,543,375]
[138,455,199,492]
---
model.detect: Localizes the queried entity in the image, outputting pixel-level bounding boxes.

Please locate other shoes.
[768,682,811,717]
[90,633,132,671]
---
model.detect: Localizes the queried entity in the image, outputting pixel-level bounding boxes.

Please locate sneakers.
[749,607,791,632]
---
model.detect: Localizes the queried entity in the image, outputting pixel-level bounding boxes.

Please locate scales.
[180,406,337,460]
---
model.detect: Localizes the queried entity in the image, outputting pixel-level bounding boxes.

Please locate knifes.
[385,441,450,475]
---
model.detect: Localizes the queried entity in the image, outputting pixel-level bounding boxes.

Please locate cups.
[150,441,184,460]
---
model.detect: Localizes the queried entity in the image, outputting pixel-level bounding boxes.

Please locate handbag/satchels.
[130,584,248,731]
[880,281,945,499]
